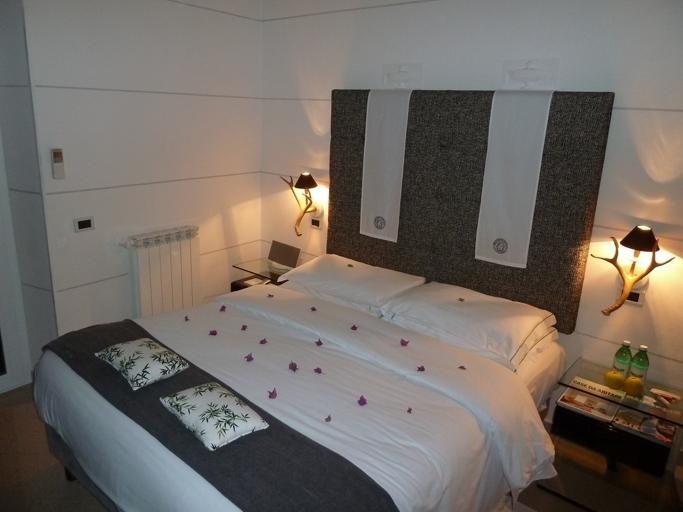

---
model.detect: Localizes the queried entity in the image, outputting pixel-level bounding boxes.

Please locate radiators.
[125,221,207,317]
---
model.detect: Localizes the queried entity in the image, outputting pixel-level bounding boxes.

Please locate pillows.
[95,335,194,393]
[159,381,268,452]
[278,249,559,371]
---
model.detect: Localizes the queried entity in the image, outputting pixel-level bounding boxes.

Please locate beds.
[27,74,619,512]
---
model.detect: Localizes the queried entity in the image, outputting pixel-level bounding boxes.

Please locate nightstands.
[541,357,682,512]
[227,255,289,292]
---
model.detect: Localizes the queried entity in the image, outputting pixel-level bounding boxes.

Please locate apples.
[623,377,643,396]
[605,370,624,389]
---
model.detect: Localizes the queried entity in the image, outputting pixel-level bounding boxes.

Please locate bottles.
[613,339,630,377]
[629,344,649,382]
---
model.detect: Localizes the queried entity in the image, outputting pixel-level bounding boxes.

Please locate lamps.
[614,224,660,290]
[292,171,323,218]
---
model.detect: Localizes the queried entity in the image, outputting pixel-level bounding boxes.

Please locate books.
[615,387,682,445]
[557,374,626,424]
